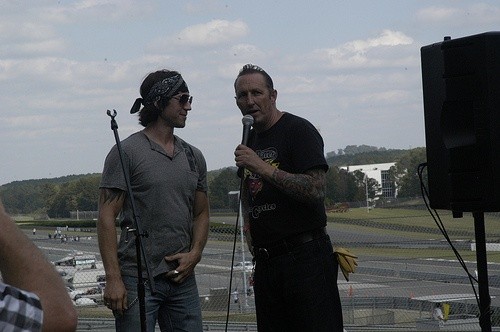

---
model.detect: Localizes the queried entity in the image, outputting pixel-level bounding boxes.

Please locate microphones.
[241,115,254,146]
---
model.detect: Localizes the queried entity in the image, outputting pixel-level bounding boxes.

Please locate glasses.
[169,94,193,105]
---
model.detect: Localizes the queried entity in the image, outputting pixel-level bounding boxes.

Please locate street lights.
[228,191,249,307]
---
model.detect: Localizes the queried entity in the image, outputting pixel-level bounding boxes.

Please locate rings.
[106,303,110,305]
[174,270,179,274]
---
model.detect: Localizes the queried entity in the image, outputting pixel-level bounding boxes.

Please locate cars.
[52,253,107,306]
[230,262,253,271]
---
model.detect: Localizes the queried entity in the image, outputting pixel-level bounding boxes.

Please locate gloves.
[334,248,359,281]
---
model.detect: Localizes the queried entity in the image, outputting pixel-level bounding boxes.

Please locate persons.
[234,288,238,303]
[234,64,344,332]
[0,201,79,332]
[432,301,450,322]
[98,69,209,332]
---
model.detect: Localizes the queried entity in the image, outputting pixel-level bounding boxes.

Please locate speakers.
[420,31,500,218]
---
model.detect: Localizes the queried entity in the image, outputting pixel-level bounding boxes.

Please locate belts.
[253,225,329,263]
